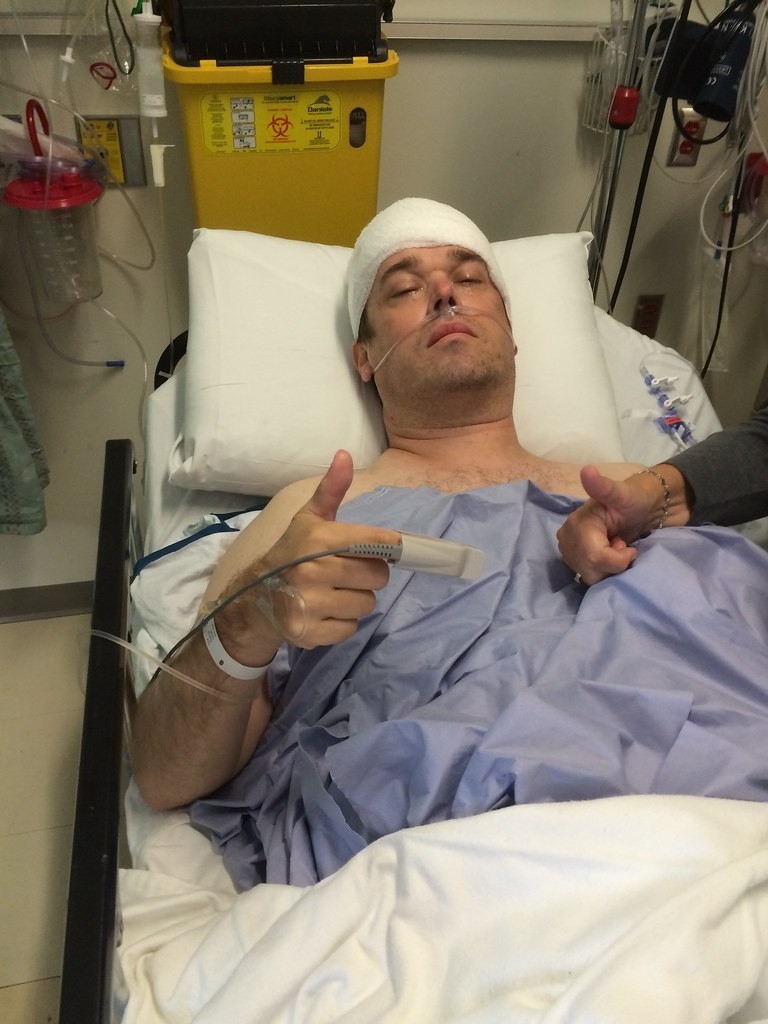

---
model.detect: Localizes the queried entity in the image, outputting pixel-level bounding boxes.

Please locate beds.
[59,304,768,1023]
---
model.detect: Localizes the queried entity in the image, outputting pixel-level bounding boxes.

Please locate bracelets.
[638,469,670,528]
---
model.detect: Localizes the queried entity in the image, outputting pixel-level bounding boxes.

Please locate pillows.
[166,227,625,497]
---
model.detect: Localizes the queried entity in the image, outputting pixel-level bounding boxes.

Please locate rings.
[574,572,581,584]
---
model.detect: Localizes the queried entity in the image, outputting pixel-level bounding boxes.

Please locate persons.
[134,194,768,811]
